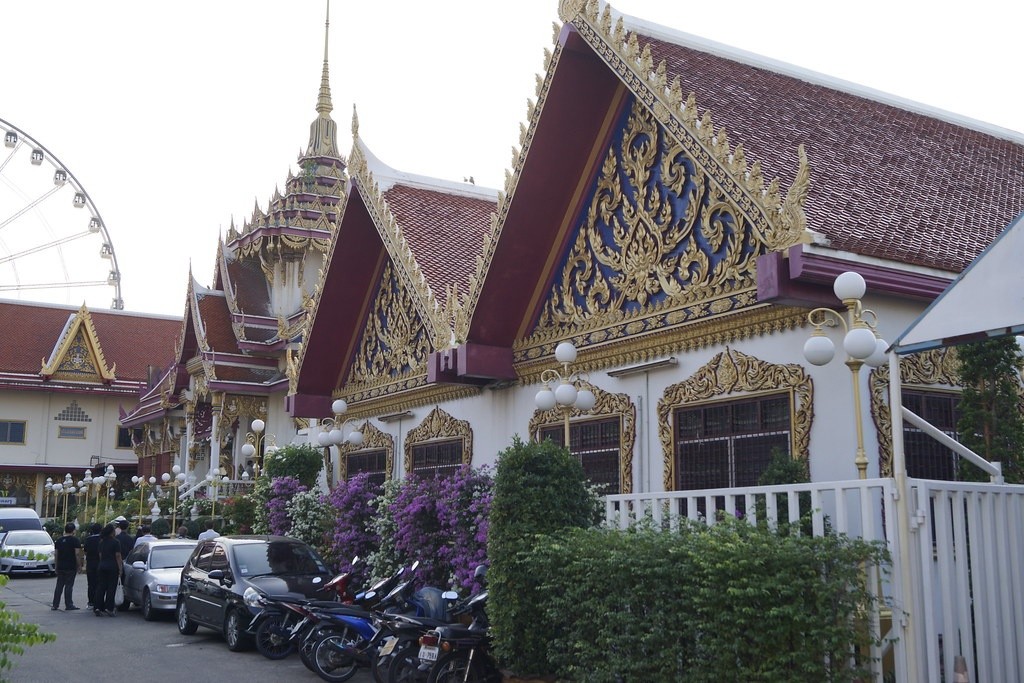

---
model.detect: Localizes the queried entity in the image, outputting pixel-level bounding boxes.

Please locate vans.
[0,507,46,532]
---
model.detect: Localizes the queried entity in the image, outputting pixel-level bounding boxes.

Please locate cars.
[119,538,200,621]
[0,529,57,576]
[176,534,355,653]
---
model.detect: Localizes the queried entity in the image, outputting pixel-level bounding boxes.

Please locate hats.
[113,515,126,524]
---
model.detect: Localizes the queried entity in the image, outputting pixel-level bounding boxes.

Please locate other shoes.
[51,605,60,610]
[94,608,104,617]
[86,601,94,607]
[65,606,80,610]
[105,608,116,617]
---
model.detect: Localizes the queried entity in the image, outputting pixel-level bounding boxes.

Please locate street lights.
[532,341,596,467]
[239,420,278,493]
[162,465,188,539]
[131,474,156,530]
[43,464,117,529]
[318,401,364,486]
[801,269,889,612]
[206,470,233,522]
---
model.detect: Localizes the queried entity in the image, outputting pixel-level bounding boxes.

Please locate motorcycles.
[247,555,495,683]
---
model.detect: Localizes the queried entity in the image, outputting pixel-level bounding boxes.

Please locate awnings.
[883,211,1024,683]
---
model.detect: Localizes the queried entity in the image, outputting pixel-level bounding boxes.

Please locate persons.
[93,524,122,617]
[134,525,158,548]
[84,523,102,610]
[115,520,134,610]
[198,520,220,541]
[175,526,190,540]
[245,460,253,475]
[51,523,82,610]
